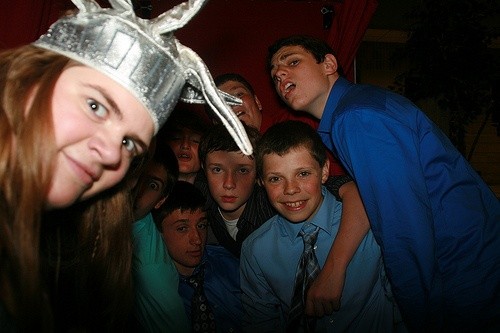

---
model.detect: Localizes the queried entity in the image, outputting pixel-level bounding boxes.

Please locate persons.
[204,74,264,131]
[0,0,252,333]
[129,148,185,333]
[240,118,401,333]
[268,32,500,333]
[170,122,203,182]
[199,122,371,319]
[150,180,241,333]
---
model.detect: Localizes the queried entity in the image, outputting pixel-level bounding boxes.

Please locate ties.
[288,226,320,326]
[186,264,216,332]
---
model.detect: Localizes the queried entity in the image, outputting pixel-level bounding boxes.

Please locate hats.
[33,0,253,154]
